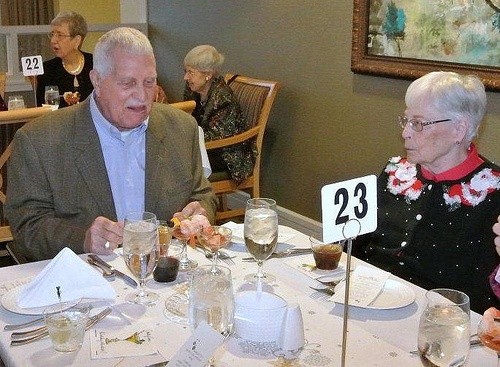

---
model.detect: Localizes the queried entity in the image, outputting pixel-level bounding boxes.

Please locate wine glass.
[195,225,233,276]
[477,307,500,367]
[122,213,159,306]
[167,215,202,271]
[243,198,279,292]
[64,89,80,106]
[188,264,234,367]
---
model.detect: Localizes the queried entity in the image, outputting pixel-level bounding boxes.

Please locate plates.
[1,285,83,315]
[334,279,415,310]
[164,290,288,344]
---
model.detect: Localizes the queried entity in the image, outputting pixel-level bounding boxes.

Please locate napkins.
[16,247,115,310]
[221,221,293,243]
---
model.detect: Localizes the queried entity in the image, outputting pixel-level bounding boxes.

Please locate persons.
[184,45,255,186]
[489,214,500,299]
[342,70,500,314]
[3,27,218,262]
[36,10,94,107]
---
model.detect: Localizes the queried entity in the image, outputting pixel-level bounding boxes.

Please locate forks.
[3,305,112,349]
[310,286,335,296]
[316,278,339,286]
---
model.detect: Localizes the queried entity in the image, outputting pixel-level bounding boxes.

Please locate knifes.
[88,254,138,287]
[242,248,313,260]
[409,339,483,355]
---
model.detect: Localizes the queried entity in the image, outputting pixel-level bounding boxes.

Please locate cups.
[43,301,89,355]
[157,220,171,255]
[8,96,24,111]
[418,288,470,367]
[308,232,346,270]
[45,86,60,107]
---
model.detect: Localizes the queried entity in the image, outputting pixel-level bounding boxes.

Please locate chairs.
[0,72,283,261]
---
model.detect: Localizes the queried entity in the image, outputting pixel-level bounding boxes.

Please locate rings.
[105,241,111,249]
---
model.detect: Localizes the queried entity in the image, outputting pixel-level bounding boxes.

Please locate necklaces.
[62,54,83,73]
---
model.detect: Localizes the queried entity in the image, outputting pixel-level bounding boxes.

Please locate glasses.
[47,33,73,40]
[397,113,452,132]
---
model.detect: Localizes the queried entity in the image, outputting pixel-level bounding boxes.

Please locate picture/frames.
[349,0,500,91]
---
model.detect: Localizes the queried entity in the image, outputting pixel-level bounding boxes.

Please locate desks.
[0,223,500,367]
